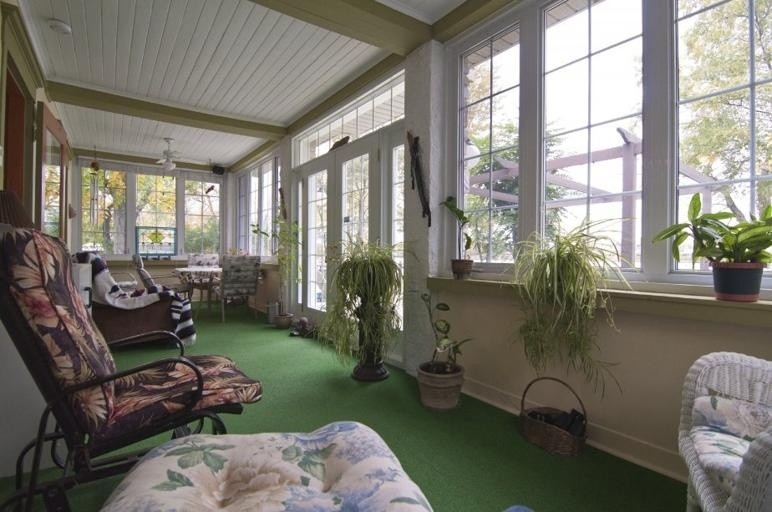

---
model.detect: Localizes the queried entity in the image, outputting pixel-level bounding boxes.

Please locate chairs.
[73,250,197,349]
[211,255,261,324]
[678,351,772,512]
[98,418,434,512]
[131,253,191,300]
[0,230,263,510]
[188,252,219,303]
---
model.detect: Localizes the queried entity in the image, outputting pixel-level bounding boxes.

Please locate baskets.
[518,377,588,456]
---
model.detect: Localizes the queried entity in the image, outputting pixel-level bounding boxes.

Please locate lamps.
[89,146,99,225]
[163,138,176,171]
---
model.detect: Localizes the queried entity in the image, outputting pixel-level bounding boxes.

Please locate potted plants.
[652,193,772,301]
[250,203,303,328]
[416,293,474,410]
[438,196,476,279]
[305,231,416,382]
